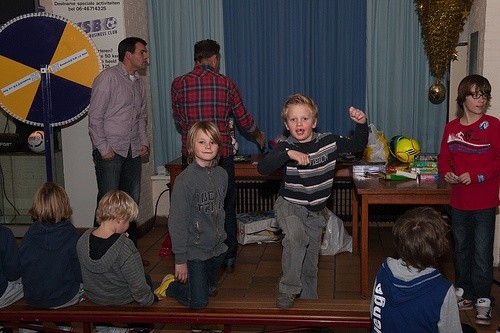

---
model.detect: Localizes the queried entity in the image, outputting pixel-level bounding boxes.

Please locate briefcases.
[235,208,284,245]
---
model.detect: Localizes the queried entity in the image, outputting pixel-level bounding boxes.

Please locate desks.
[165,157,351,195]
[351,173,450,299]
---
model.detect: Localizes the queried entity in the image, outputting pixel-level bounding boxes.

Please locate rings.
[144,154,145,157]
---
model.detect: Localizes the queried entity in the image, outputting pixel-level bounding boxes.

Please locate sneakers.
[154,273,177,295]
[474,297,495,325]
[454,287,474,310]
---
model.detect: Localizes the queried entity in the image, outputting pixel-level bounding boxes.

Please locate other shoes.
[221,264,235,274]
[275,292,296,308]
[28,320,73,333]
[95,323,156,333]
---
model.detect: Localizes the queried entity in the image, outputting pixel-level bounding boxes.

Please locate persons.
[437,74,500,324]
[76,191,167,333]
[19,183,89,332]
[0,227,24,309]
[87,37,154,266]
[155,121,229,309]
[370,206,477,333]
[256,95,368,333]
[171,39,261,271]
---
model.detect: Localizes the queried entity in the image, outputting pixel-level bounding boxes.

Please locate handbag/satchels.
[362,123,390,163]
[320,207,353,256]
[159,234,173,257]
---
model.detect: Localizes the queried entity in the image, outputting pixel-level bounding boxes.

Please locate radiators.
[235,189,350,215]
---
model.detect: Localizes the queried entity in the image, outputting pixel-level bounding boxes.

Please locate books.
[352,162,386,173]
[409,153,439,176]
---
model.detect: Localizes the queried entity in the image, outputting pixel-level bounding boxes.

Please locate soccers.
[395,135,421,164]
[27,130,46,154]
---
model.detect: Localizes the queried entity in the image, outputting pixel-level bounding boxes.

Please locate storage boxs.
[414,152,439,175]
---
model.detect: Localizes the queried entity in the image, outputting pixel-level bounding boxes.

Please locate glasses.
[467,92,489,99]
